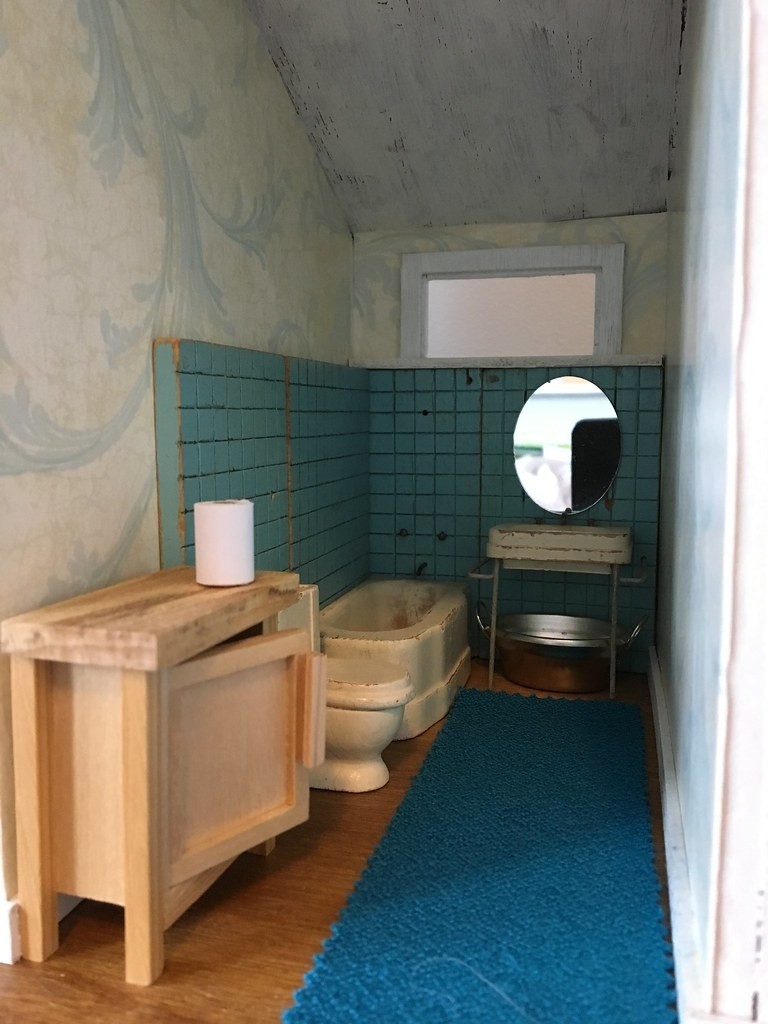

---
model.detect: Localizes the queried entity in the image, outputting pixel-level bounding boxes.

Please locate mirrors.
[511,375,622,514]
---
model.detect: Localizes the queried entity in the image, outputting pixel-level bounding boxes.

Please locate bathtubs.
[318,573,471,739]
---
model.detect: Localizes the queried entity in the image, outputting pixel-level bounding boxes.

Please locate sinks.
[485,522,633,564]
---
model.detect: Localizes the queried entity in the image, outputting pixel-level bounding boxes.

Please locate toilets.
[309,656,416,793]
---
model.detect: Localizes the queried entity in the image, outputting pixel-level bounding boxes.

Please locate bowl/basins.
[476,600,648,693]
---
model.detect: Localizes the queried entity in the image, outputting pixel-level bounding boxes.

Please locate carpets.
[277,684,681,1023]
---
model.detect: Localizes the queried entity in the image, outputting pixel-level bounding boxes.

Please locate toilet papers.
[192,497,255,587]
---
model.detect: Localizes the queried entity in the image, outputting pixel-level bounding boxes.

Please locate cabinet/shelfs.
[0,565,327,989]
